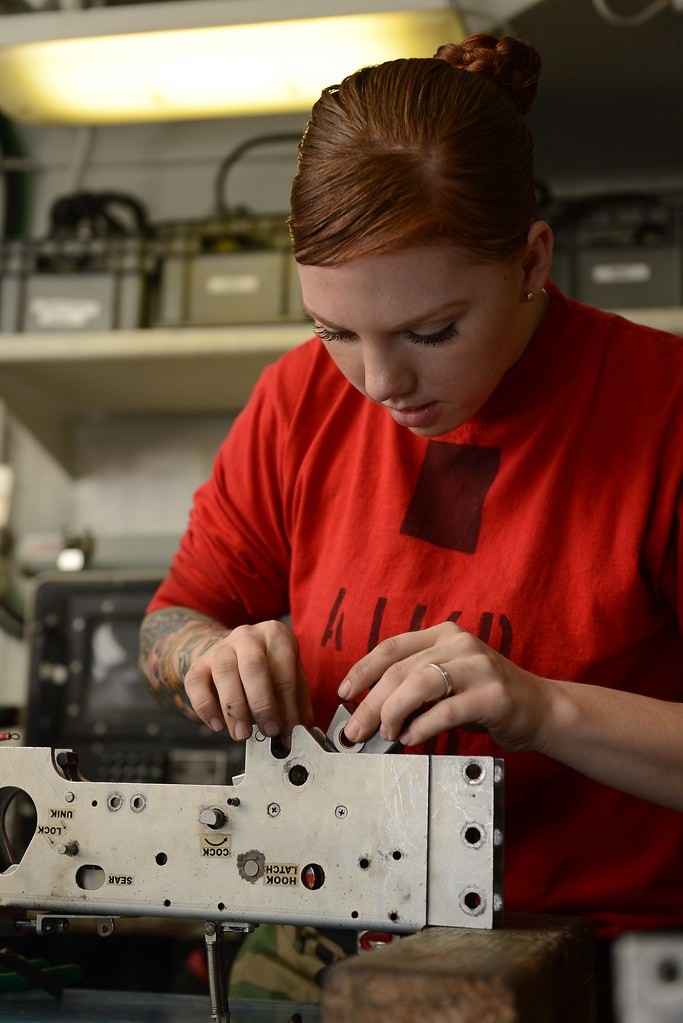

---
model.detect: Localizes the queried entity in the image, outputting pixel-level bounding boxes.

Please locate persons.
[139,33,683,1023]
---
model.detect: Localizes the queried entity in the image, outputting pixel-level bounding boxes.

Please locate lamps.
[0,1,539,124]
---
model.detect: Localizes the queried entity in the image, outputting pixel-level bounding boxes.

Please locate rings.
[426,662,453,699]
